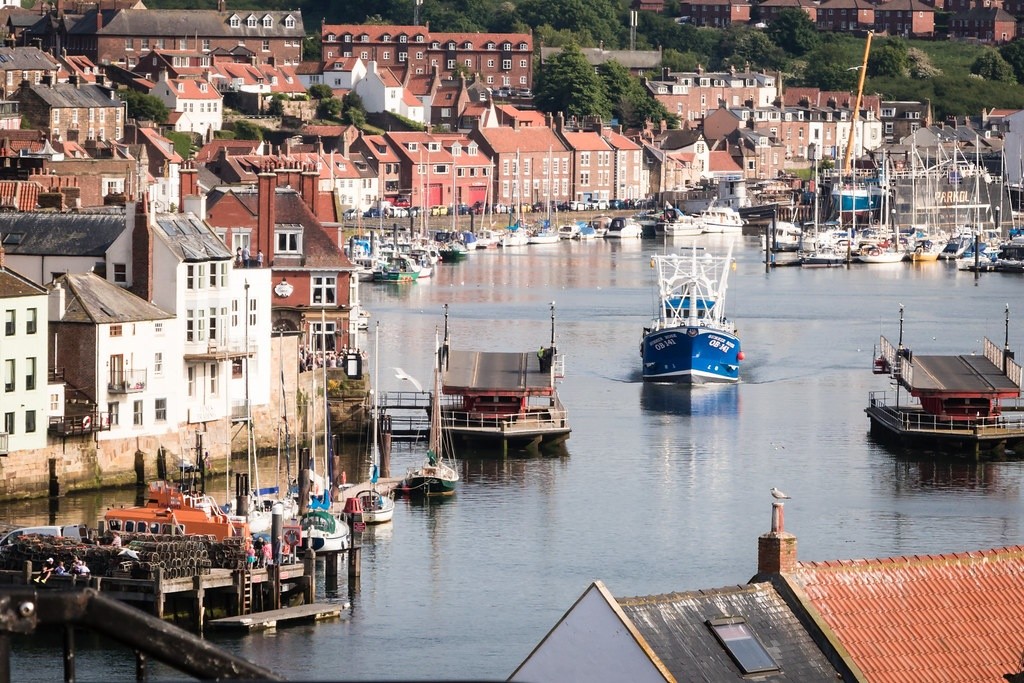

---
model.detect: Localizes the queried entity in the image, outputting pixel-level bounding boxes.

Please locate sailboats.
[104,306,460,557]
[344,29,1024,285]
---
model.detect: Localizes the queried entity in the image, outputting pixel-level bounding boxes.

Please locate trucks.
[0,523,89,555]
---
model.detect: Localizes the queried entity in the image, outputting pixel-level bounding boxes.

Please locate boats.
[358,304,370,331]
[639,240,745,385]
